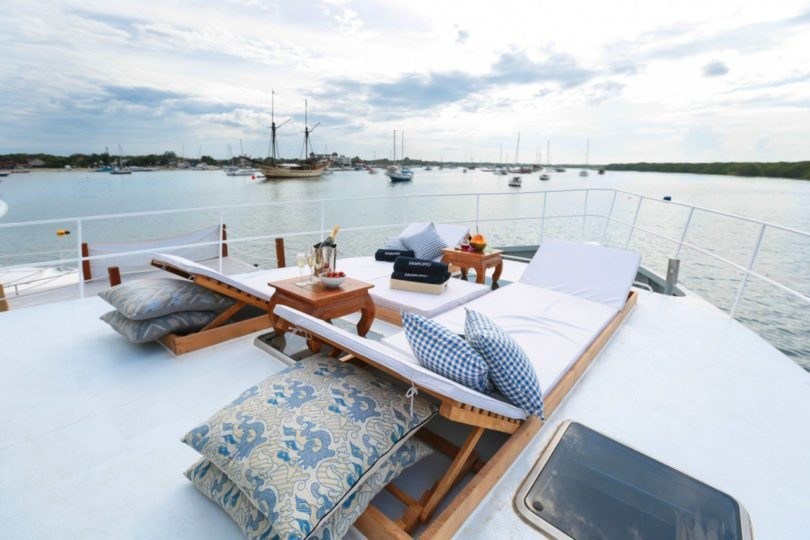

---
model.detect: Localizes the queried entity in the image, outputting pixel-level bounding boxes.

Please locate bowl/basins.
[320,271,346,288]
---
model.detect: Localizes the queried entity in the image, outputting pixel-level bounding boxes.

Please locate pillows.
[400,310,490,391]
[97,279,235,345]
[384,236,407,251]
[182,355,439,540]
[399,222,447,261]
[183,439,433,539]
[464,307,544,423]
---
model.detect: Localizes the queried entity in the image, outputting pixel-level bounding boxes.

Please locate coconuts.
[470,234,486,251]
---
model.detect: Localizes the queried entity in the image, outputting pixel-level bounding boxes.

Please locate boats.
[94,142,152,175]
[509,176,522,187]
[0,167,30,176]
[350,128,456,183]
[578,138,589,176]
[540,175,549,180]
[556,167,565,173]
[467,132,546,176]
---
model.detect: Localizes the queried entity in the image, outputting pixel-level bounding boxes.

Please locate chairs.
[154,221,469,314]
[274,238,642,540]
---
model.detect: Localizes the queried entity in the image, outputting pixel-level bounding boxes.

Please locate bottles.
[318,224,340,247]
[294,254,308,287]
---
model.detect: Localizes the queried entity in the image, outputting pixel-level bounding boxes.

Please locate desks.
[443,244,505,288]
[268,274,377,353]
[361,275,488,327]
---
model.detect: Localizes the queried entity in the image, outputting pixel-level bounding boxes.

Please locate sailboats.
[177,87,333,180]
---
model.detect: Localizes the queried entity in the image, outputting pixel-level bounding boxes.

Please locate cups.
[461,239,469,252]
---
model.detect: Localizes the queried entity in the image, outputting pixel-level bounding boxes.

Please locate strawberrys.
[324,271,345,278]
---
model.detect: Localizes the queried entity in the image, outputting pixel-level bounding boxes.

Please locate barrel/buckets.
[312,243,336,282]
